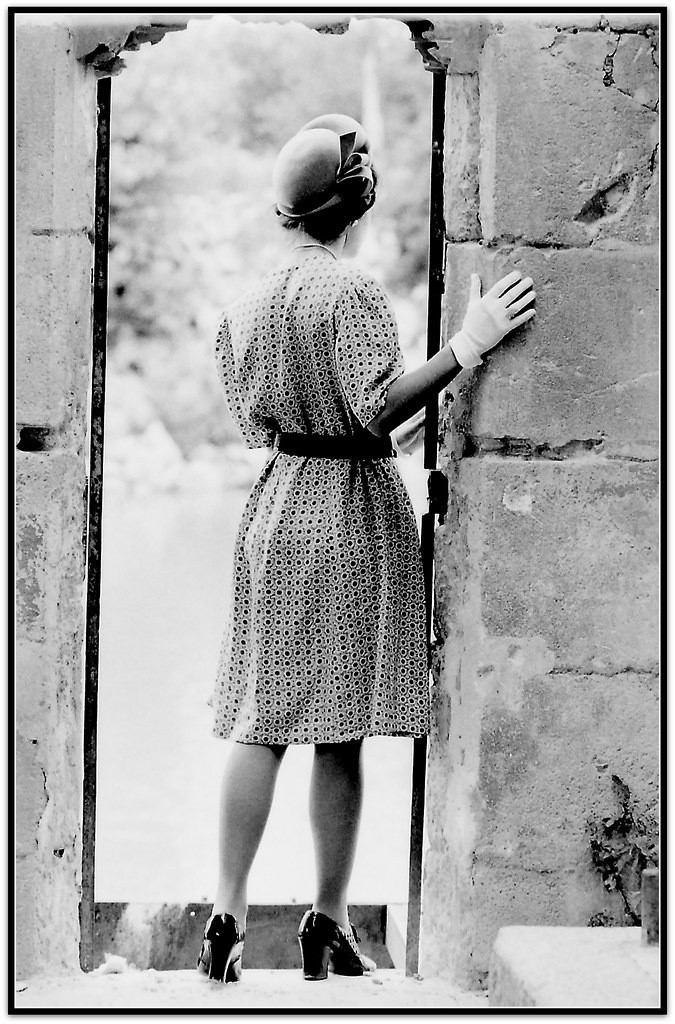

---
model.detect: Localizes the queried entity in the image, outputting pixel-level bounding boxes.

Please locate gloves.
[448,270,539,369]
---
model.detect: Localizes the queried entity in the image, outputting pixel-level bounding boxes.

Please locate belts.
[270,432,394,462]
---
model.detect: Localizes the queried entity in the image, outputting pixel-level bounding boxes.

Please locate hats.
[270,113,375,218]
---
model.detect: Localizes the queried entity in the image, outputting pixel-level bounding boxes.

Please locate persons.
[196,113,538,982]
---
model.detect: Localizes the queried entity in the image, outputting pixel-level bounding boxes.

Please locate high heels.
[296,910,380,981]
[195,915,246,985]
